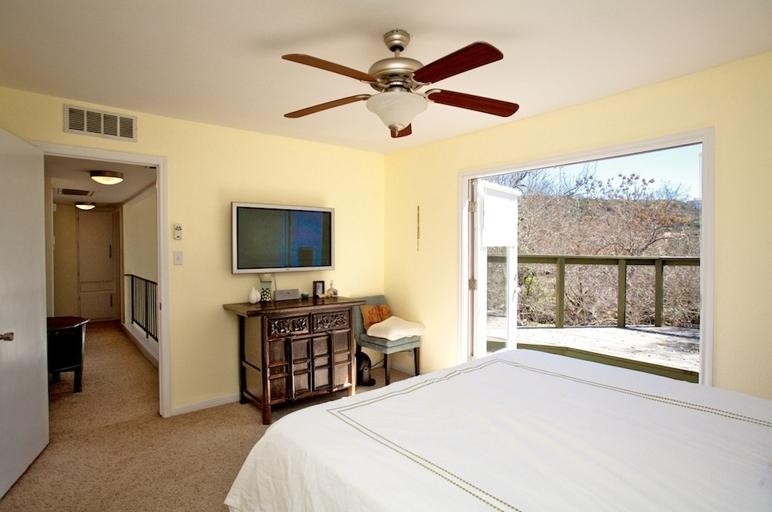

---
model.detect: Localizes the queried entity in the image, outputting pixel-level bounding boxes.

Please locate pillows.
[358,303,392,331]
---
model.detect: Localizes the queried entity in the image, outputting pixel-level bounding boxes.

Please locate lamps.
[74,201,99,212]
[362,84,430,133]
[86,168,127,188]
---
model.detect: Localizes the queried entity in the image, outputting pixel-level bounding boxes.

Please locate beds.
[219,336,770,512]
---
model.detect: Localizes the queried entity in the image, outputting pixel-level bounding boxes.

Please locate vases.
[248,286,259,305]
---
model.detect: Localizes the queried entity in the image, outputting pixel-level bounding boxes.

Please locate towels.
[366,315,424,340]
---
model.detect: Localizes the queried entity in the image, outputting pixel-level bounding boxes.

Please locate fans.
[274,28,523,138]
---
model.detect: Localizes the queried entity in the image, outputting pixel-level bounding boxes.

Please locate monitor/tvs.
[231,202,335,274]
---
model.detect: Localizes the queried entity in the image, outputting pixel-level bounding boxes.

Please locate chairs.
[350,294,425,386]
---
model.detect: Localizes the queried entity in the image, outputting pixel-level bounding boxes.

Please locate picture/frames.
[311,281,326,299]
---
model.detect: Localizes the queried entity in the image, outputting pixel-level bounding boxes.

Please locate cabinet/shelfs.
[221,292,369,426]
[47,312,95,394]
